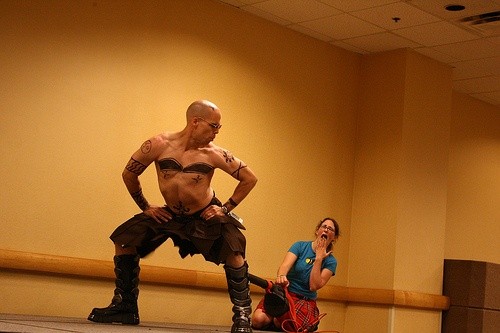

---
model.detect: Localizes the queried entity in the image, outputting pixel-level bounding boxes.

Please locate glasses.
[198,117,222,130]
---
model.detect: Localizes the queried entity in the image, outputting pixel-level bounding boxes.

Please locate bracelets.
[229,197,237,208]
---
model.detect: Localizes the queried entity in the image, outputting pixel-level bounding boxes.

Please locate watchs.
[221,205,229,216]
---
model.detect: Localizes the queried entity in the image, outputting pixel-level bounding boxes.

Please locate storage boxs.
[441,259,500,333]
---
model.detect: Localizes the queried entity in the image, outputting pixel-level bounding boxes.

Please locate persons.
[251,216,340,333]
[87,100,259,333]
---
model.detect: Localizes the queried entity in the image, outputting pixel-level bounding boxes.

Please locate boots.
[88,254,140,325]
[223,260,253,333]
